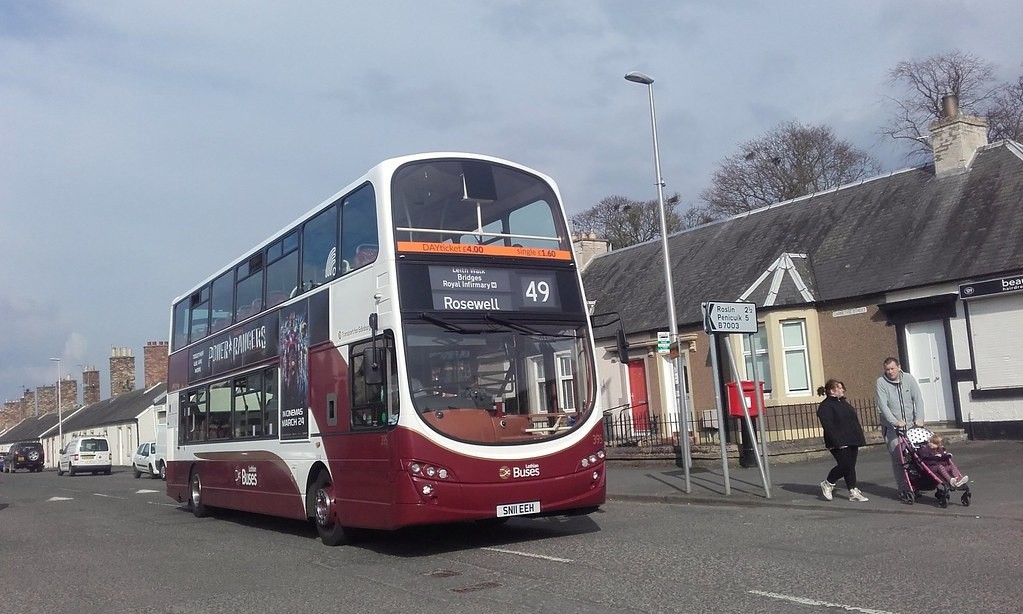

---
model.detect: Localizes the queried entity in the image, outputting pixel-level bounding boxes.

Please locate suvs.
[56,435,112,477]
[3,442,45,473]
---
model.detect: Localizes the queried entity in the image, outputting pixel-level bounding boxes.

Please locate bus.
[159,148,631,547]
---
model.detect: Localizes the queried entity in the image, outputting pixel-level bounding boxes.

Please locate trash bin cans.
[602,411,614,440]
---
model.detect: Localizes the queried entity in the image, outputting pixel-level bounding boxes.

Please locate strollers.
[894,423,973,508]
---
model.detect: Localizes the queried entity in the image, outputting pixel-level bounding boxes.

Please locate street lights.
[623,72,694,470]
[49,357,63,454]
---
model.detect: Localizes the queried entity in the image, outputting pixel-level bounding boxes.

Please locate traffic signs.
[701,300,759,335]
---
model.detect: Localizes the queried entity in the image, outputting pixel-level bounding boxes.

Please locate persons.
[817,379,869,502]
[919,432,969,488]
[875,357,925,500]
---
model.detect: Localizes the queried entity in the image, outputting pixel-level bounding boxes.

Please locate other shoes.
[849,487,869,502]
[820,480,836,500]
[898,492,922,501]
[950,478,957,488]
[956,476,969,487]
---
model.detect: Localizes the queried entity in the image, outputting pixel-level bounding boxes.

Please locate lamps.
[689,341,697,352]
[610,352,618,363]
[647,347,654,358]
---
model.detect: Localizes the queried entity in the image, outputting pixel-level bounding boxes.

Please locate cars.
[132,441,166,480]
[0,451,9,472]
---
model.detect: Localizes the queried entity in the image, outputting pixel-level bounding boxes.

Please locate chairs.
[252,299,262,315]
[214,317,226,332]
[238,305,252,321]
[355,243,378,269]
[91,445,99,450]
[260,399,277,434]
[267,290,290,308]
